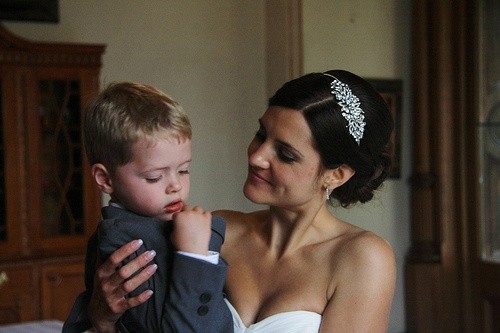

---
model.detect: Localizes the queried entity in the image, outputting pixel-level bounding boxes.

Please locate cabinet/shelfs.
[0,23,111,325]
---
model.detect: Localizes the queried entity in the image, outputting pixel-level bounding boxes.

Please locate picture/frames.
[364,72,404,182]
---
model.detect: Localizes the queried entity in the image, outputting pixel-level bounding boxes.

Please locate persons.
[63,70,399,333]
[85,80,234,333]
